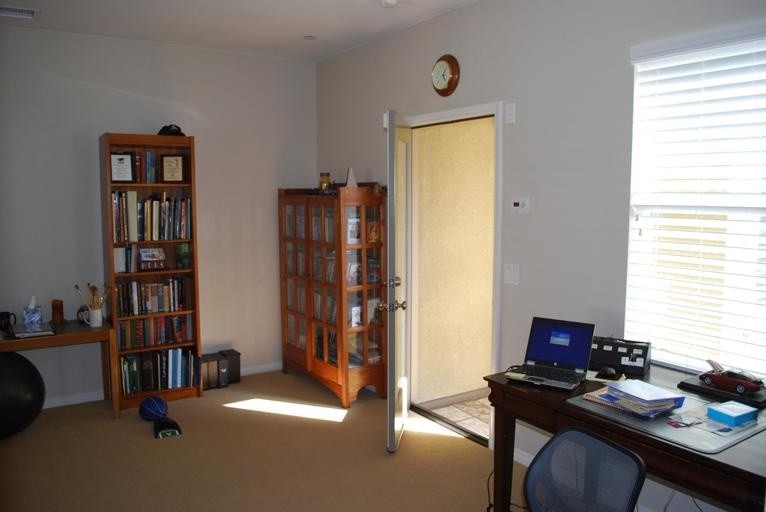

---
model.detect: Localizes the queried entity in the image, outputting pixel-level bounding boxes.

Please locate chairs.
[522,427,647,512]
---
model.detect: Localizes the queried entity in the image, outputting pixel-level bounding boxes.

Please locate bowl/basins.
[76,310,88,322]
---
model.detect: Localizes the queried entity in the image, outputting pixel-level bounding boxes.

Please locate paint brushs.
[74,284,111,310]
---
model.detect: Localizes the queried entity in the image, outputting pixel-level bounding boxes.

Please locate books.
[287,209,383,368]
[583,386,666,419]
[107,146,199,395]
[10,323,56,338]
[676,373,766,408]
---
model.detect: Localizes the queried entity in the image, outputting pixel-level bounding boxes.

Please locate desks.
[1,314,121,423]
[484,352,766,512]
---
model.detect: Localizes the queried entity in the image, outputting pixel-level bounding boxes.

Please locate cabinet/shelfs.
[97,132,204,418]
[277,186,387,404]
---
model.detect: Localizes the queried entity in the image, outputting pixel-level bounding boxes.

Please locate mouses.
[599,367,616,377]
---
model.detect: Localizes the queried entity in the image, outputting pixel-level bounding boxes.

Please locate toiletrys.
[21,295,44,332]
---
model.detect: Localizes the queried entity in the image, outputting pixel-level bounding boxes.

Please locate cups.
[50,300,63,324]
[82,308,102,328]
[0,312,16,333]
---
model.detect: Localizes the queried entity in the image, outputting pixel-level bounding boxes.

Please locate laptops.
[504,316,595,390]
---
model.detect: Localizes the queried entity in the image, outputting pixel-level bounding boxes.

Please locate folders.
[598,379,685,418]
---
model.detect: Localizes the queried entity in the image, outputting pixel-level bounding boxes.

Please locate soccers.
[139,396,167,421]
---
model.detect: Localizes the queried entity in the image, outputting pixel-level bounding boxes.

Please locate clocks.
[430,55,460,96]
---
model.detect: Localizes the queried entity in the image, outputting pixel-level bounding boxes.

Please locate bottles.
[318,172,330,192]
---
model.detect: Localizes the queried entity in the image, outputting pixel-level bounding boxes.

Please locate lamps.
[0,7,35,21]
